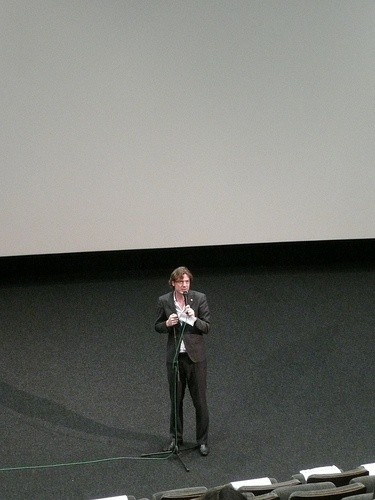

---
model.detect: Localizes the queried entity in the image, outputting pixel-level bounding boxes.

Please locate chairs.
[98,461,375,500]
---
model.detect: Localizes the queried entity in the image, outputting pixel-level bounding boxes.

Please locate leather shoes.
[163,440,182,452]
[199,443,209,457]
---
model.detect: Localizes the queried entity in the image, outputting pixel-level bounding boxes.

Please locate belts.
[178,352,189,358]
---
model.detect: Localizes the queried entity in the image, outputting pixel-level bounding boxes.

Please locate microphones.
[183,291,188,307]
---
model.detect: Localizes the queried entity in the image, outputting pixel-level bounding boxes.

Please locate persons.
[155,266,211,458]
